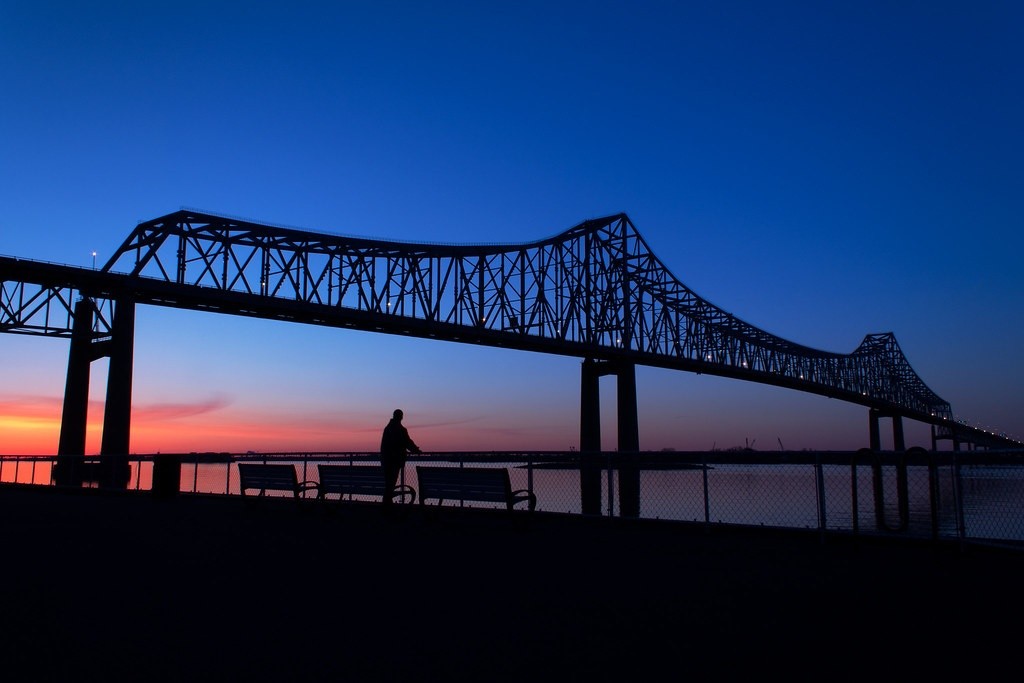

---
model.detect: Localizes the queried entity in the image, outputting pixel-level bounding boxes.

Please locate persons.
[380,408,422,518]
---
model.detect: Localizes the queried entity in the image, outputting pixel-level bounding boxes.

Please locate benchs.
[315,464,415,511]
[416,465,538,517]
[237,461,320,504]
